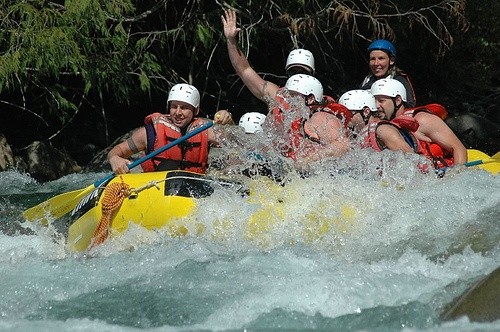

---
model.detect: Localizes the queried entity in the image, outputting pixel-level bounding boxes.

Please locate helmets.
[286,49,315,76]
[285,74,324,103]
[339,89,377,111]
[371,75,407,102]
[367,39,395,59]
[167,84,200,115]
[238,112,267,134]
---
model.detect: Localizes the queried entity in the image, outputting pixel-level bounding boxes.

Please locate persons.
[109,83,234,175]
[210,9,469,183]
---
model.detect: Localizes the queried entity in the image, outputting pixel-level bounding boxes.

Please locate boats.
[65,149,500,248]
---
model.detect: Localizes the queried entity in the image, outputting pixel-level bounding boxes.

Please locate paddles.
[23,115,221,226]
[435,150,500,172]
